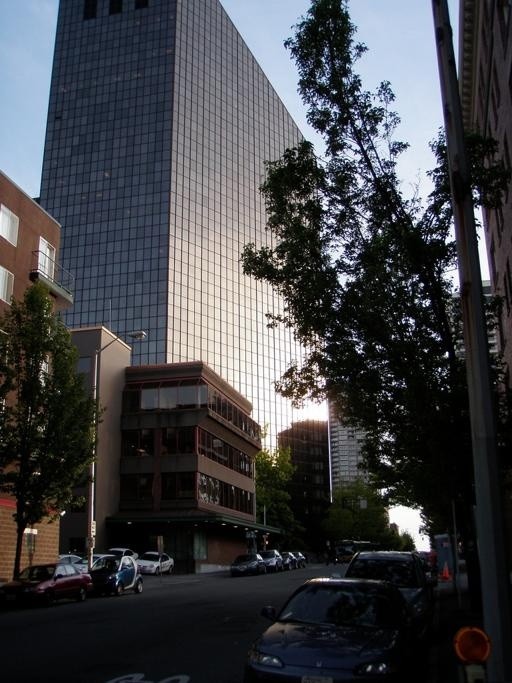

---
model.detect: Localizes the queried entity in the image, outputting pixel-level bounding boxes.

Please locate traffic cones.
[441,561,453,582]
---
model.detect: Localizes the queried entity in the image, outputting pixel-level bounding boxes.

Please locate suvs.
[259,549,284,573]
[330,550,439,636]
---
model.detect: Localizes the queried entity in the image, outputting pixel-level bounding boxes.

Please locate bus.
[335,540,372,563]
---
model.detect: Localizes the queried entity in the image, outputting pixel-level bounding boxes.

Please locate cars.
[0,561,93,609]
[72,554,116,574]
[231,553,267,577]
[90,555,144,596]
[280,552,299,571]
[292,552,306,568]
[58,553,83,564]
[135,551,175,576]
[244,576,420,682]
[107,548,139,566]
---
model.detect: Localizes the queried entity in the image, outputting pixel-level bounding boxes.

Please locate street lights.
[87,331,148,569]
